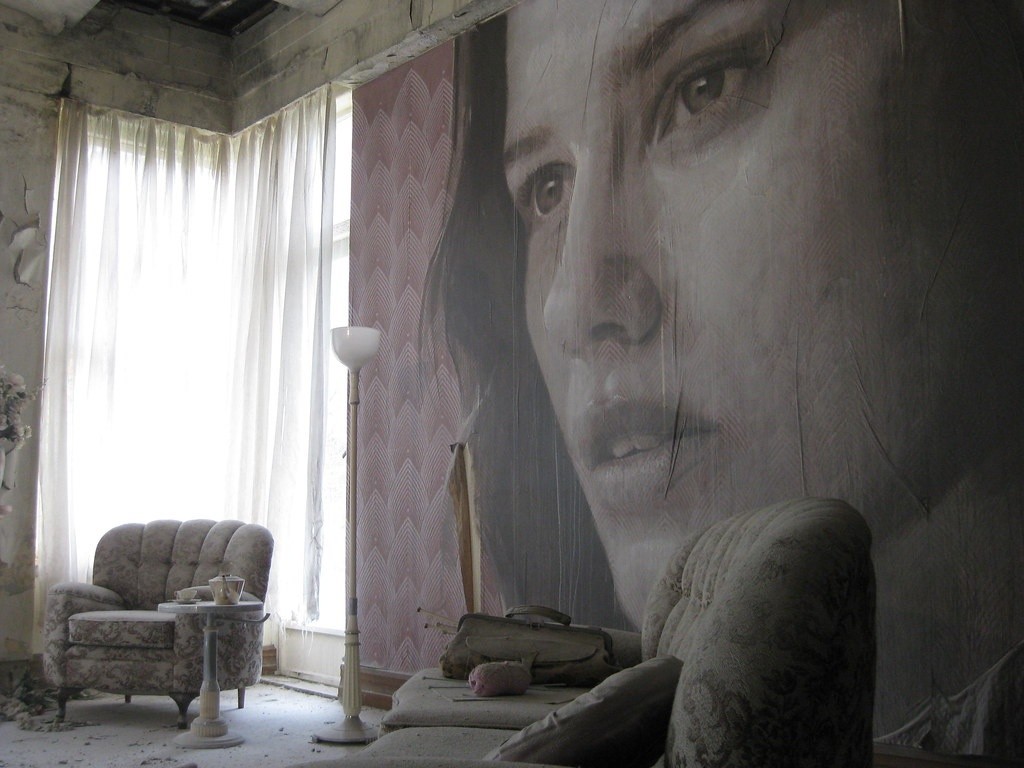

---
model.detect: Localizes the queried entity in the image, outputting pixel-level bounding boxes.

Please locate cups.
[174,589,197,600]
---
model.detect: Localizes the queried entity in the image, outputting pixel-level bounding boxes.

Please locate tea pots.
[209,574,245,605]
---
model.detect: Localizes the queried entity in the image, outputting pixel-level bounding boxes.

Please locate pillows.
[482,654,683,768]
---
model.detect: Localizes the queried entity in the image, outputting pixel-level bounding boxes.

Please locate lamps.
[311,326,378,745]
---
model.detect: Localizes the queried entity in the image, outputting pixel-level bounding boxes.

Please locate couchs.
[41,519,275,731]
[284,496,877,768]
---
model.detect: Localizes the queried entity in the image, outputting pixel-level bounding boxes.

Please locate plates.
[173,598,202,604]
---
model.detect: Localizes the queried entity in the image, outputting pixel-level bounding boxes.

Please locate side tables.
[156,602,265,750]
